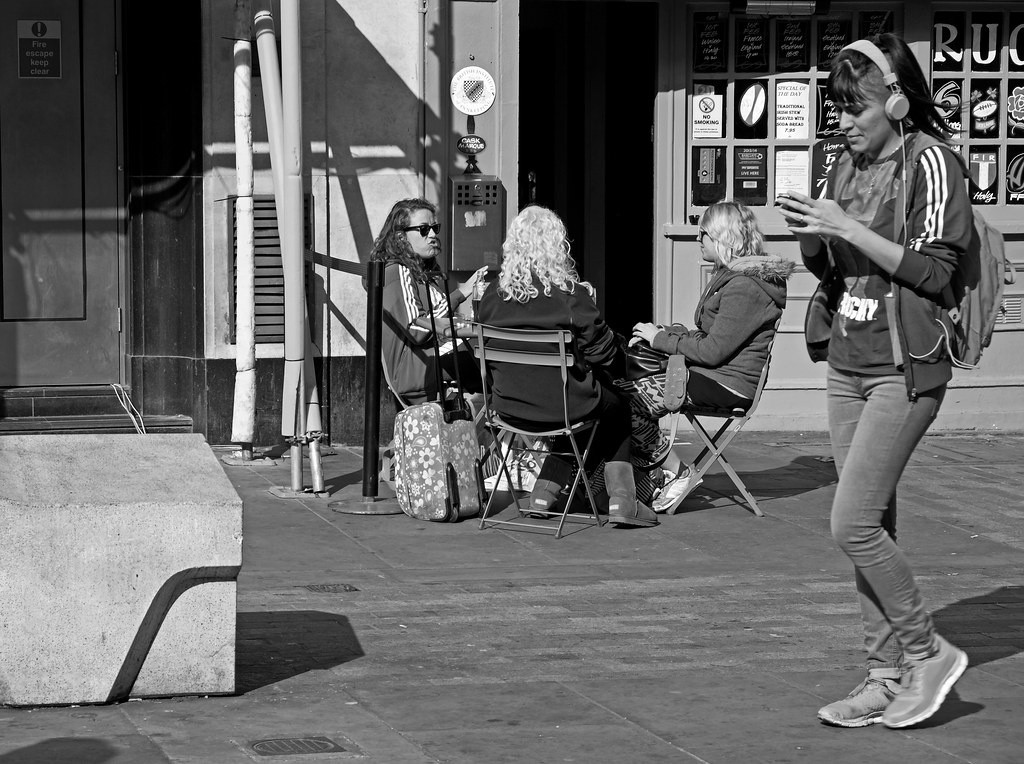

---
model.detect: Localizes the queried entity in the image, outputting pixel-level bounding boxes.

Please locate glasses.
[403,224,441,237]
[698,230,708,241]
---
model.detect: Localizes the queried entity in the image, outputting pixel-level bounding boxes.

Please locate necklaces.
[865,146,900,194]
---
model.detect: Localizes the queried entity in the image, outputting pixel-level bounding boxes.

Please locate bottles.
[472,271,485,319]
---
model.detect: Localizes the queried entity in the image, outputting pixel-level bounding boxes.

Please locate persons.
[612,201,797,513]
[471,205,658,528]
[773,33,978,733]
[362,196,547,494]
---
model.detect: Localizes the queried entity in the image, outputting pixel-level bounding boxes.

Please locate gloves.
[664,354,687,410]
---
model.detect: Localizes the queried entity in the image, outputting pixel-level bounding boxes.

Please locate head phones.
[840,39,910,120]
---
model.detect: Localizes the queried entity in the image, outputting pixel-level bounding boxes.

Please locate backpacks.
[891,137,1016,370]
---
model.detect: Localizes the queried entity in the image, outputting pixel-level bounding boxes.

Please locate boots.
[529,455,571,518]
[604,460,659,527]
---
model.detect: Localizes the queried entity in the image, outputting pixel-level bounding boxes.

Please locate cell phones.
[779,193,807,216]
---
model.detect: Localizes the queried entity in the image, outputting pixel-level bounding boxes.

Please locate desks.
[415,316,483,371]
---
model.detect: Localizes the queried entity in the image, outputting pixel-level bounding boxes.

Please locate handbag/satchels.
[622,323,690,381]
[585,457,663,512]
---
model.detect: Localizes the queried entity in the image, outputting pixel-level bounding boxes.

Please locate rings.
[800,214,806,225]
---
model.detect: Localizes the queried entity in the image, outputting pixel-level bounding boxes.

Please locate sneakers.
[883,637,968,728]
[817,662,901,728]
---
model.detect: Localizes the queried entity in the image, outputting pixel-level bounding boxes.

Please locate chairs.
[461,321,606,540]
[661,317,783,515]
[377,344,546,474]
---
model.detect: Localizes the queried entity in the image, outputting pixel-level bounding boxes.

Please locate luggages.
[382,271,489,522]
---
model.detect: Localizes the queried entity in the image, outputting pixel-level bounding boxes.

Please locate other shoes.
[651,464,703,512]
